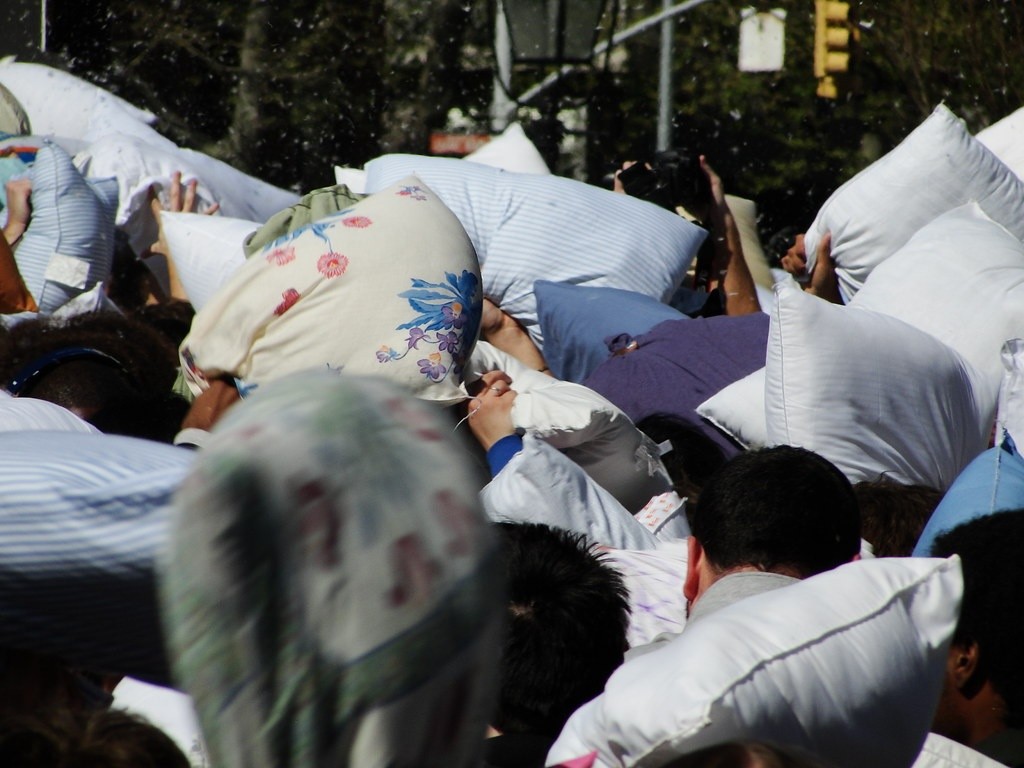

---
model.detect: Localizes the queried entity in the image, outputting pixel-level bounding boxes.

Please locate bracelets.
[727,289,740,296]
[537,366,549,373]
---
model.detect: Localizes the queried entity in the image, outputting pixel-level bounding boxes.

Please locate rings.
[490,387,502,393]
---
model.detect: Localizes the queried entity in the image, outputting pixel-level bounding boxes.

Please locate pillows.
[0,54,1024,767]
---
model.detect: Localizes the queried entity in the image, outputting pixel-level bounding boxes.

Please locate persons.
[0,153,1024,768]
